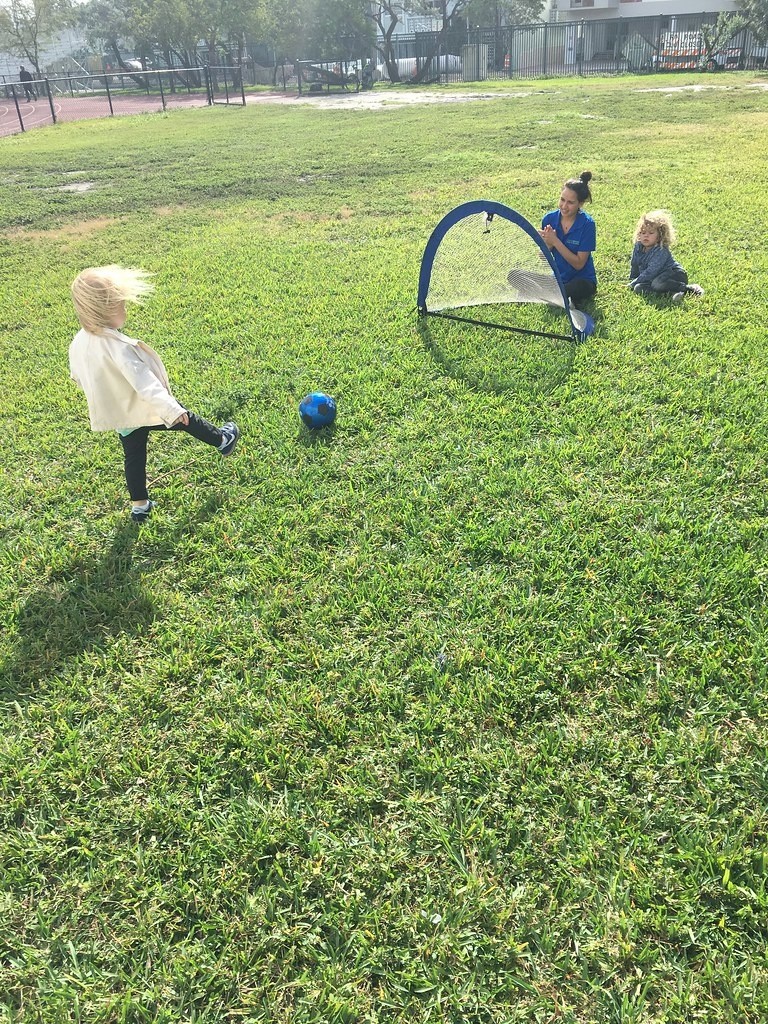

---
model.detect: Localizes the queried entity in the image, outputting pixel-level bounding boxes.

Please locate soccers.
[299,392,337,430]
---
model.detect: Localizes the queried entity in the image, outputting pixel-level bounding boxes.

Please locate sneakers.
[131,500,156,520]
[219,422,240,456]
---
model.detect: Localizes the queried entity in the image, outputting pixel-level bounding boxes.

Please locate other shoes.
[672,291,684,301]
[693,284,705,295]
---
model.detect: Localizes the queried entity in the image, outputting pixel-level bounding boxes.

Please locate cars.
[652,43,727,71]
[116,57,153,79]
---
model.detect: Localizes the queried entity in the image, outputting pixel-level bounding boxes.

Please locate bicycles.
[345,65,373,92]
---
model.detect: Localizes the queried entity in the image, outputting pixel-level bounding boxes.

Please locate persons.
[68,267,239,521]
[507,172,596,309]
[622,211,705,300]
[20,66,37,102]
